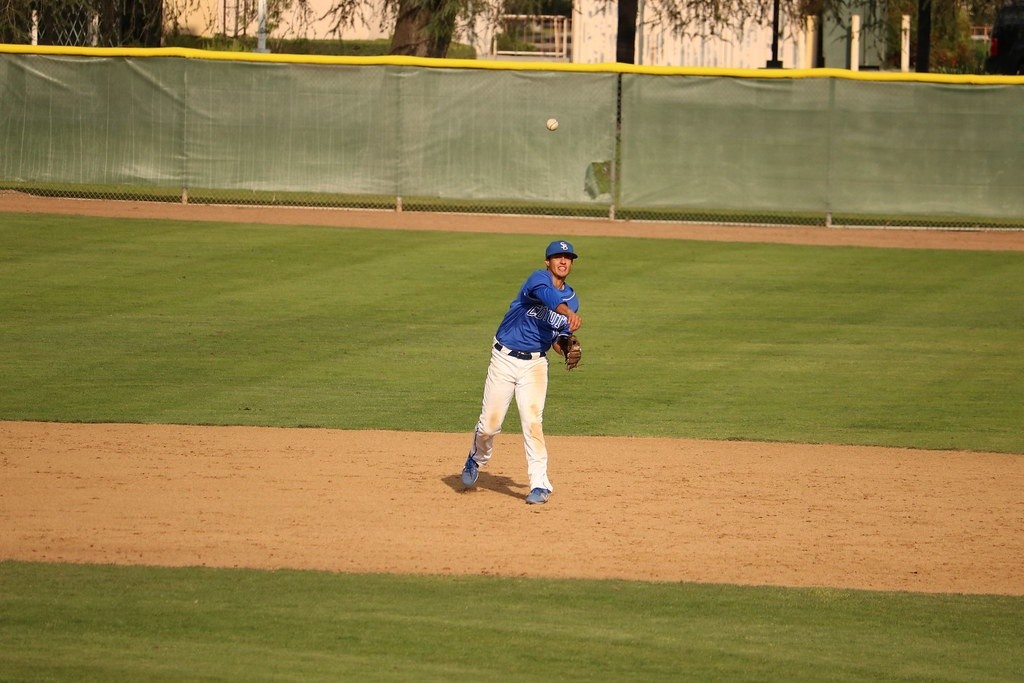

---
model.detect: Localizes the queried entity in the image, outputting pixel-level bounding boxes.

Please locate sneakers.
[526,487,548,504]
[462,456,479,488]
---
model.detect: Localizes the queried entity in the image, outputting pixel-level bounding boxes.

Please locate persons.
[461,240,583,506]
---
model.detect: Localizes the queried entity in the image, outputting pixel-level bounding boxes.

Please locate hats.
[546,241,578,259]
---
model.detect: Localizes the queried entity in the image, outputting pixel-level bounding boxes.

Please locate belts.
[495,342,546,359]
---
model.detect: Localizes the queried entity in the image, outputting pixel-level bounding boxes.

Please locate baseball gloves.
[558,334,582,370]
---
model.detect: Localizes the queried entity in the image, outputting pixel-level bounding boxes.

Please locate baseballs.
[546,118,559,131]
